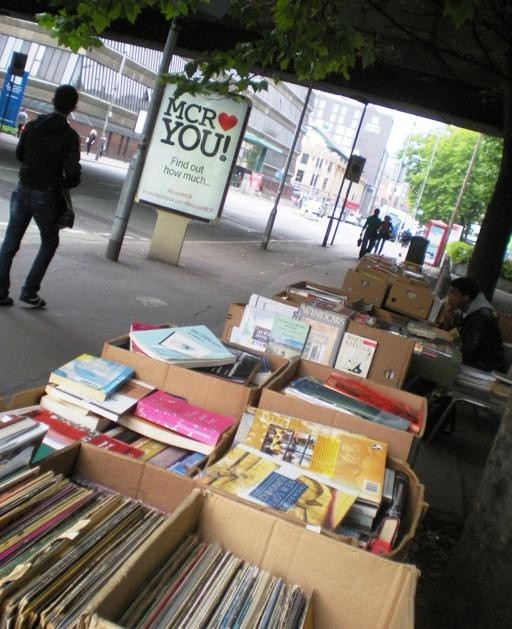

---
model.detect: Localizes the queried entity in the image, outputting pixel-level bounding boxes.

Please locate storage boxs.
[0,252,453,626]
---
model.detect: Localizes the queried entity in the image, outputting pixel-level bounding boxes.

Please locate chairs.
[425,343,512,448]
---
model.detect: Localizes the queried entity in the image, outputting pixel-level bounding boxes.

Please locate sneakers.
[19,293,47,308]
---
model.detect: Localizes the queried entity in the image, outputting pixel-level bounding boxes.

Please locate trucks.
[382,212,410,246]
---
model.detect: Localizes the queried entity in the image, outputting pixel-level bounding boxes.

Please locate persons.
[16,106,28,137]
[357,207,381,258]
[0,84,83,308]
[373,215,392,255]
[95,133,107,160]
[85,128,98,155]
[399,227,412,248]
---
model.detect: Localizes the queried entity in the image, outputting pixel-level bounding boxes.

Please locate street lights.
[406,125,452,236]
[302,122,351,248]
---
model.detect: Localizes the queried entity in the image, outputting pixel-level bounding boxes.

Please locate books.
[0,281,414,629]
[347,250,512,406]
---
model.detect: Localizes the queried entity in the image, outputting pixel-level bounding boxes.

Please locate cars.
[289,188,369,229]
